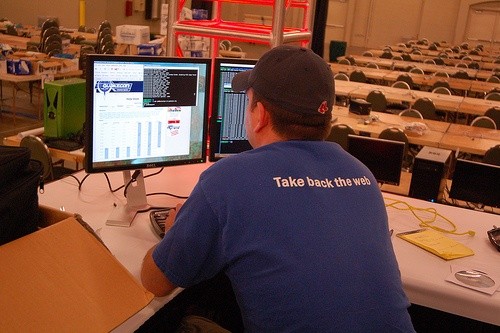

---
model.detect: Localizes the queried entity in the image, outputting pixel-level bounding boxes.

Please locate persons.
[140,45,415,333]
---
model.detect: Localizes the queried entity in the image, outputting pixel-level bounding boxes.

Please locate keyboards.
[150,207,176,240]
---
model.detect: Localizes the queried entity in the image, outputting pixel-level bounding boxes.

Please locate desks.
[415,64,478,79]
[327,63,392,85]
[334,79,365,106]
[476,70,494,79]
[384,70,471,97]
[471,80,500,98]
[2,136,220,333]
[349,84,464,123]
[367,50,425,62]
[482,57,500,63]
[482,62,500,70]
[0,27,140,127]
[336,55,411,70]
[411,55,480,68]
[331,99,500,327]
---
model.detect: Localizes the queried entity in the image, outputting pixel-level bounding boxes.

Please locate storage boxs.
[117,24,151,46]
[44,79,87,137]
[0,206,156,333]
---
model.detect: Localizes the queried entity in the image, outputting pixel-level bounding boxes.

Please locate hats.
[233,45,335,116]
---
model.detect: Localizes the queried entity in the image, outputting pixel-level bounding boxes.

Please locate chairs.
[327,37,500,171]
[25,15,116,104]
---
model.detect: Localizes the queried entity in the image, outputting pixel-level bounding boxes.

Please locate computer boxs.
[409,146,453,203]
[43,77,86,140]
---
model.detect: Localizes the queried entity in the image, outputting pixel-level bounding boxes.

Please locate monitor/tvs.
[448,158,500,212]
[346,134,405,186]
[83,53,257,225]
